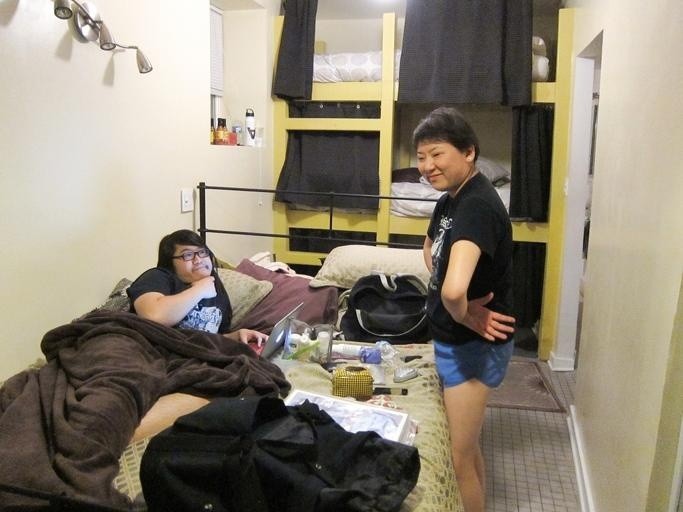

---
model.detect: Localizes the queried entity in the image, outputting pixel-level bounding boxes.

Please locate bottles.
[244,107,254,146]
[211,118,242,146]
[332,343,362,358]
[375,340,405,369]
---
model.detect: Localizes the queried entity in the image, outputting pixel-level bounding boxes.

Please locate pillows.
[207,244,442,340]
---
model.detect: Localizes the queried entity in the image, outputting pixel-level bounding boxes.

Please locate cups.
[358,345,381,364]
[288,327,329,357]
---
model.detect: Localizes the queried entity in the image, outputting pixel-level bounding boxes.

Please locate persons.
[126,229,271,347]
[412,104,522,511]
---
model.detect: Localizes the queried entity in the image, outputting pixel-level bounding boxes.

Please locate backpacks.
[336,270,434,344]
[137,389,420,511]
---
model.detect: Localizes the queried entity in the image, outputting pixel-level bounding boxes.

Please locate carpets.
[484,362,566,412]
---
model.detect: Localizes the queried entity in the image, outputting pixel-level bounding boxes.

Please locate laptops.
[248,301,306,359]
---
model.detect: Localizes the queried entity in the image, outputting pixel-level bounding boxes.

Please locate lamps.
[48,0,155,75]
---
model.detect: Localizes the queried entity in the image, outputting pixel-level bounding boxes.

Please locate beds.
[1,183,469,512]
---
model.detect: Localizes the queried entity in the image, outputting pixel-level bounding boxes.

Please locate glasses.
[168,247,210,261]
